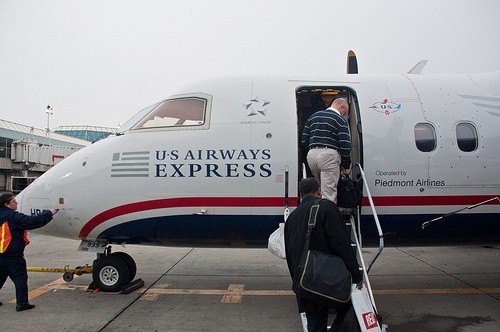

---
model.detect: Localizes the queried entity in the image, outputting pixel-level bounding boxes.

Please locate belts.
[309,145,336,150]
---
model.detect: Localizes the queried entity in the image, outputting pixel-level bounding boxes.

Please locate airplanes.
[15,50,500,332]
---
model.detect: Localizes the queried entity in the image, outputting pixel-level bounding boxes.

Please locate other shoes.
[16,303,35,311]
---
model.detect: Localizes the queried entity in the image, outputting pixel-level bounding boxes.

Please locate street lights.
[46,105,54,133]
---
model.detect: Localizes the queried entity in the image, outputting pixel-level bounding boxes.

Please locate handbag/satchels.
[338,172,363,209]
[267,208,291,259]
[350,282,382,332]
[292,198,352,311]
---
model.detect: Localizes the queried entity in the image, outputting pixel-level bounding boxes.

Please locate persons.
[302,98,352,204]
[284,177,363,332]
[0,193,59,312]
